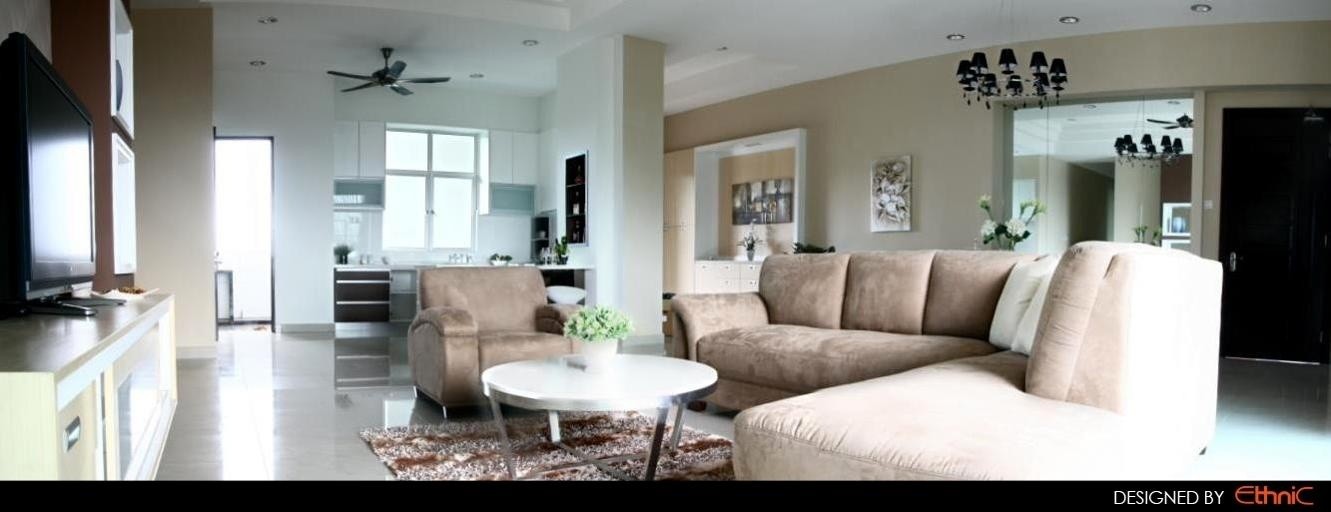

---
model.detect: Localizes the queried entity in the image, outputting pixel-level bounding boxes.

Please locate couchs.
[406,266,563,421]
[668,240,1227,483]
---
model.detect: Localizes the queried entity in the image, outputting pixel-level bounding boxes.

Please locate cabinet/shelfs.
[333,266,392,322]
[3,290,181,483]
[48,2,138,290]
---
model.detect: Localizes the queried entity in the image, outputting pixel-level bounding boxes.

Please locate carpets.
[361,417,735,481]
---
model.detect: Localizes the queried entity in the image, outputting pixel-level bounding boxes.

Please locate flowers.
[971,196,1043,250]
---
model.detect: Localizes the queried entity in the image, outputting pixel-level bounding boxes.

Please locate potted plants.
[559,306,631,359]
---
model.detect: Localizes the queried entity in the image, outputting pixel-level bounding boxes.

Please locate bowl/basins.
[547,287,586,305]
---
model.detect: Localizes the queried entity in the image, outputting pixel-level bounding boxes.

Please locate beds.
[486,352,711,482]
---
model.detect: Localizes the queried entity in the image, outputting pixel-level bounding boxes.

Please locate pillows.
[984,253,1053,350]
[1009,255,1062,355]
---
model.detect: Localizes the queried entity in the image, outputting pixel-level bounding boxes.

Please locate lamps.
[1114,97,1189,172]
[955,1,1065,111]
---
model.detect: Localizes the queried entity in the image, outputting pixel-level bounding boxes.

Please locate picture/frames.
[869,158,913,239]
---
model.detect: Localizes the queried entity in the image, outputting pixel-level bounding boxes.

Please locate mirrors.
[998,89,1200,256]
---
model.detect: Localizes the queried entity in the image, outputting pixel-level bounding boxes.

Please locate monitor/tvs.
[0,32,127,316]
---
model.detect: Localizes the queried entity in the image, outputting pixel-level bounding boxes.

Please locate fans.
[326,45,449,99]
[1145,114,1200,138]
